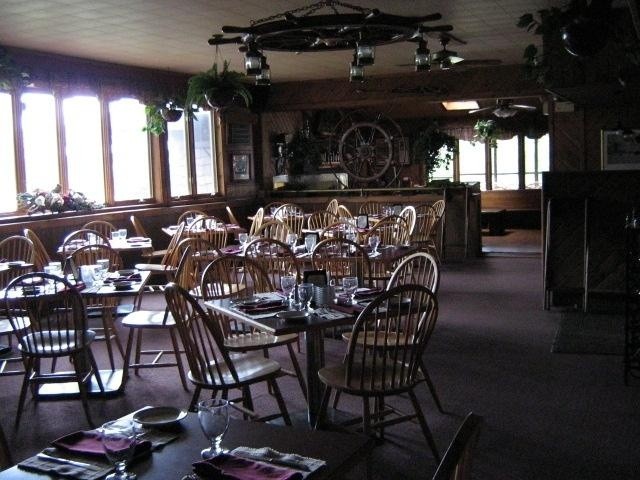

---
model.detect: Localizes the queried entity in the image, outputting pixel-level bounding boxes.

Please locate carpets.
[552,308,625,356]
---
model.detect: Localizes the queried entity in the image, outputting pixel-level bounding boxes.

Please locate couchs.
[479,188,541,227]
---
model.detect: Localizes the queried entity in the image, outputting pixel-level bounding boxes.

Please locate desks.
[481,207,508,233]
[1,408,371,480]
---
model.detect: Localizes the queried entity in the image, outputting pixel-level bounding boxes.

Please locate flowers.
[14,182,100,216]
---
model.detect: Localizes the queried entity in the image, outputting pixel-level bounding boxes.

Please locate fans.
[466,98,537,120]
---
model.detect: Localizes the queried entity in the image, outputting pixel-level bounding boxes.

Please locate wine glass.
[278,276,314,311]
[342,275,359,307]
[45,260,110,291]
[237,232,316,254]
[100,420,136,480]
[197,399,232,459]
[344,235,380,259]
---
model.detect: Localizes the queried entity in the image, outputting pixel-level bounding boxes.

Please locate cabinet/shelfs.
[541,169,640,316]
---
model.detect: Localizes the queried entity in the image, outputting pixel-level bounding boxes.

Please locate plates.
[383,297,412,307]
[113,281,133,288]
[119,270,134,276]
[230,294,284,308]
[133,406,190,425]
[20,286,40,293]
[278,311,309,320]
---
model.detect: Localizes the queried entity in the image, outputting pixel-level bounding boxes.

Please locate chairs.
[166,282,288,424]
[433,410,483,479]
[0,197,444,401]
[11,271,103,430]
[315,284,440,459]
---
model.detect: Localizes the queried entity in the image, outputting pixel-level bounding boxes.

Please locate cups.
[85,228,127,248]
[186,216,217,231]
[315,287,329,307]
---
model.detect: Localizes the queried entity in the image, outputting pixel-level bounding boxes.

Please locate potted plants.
[182,58,249,114]
[140,85,198,136]
[0,44,31,96]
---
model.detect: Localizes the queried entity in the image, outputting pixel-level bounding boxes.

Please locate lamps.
[494,107,520,120]
[208,0,459,84]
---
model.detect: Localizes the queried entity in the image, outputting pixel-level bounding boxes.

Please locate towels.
[192,451,304,480]
[50,430,153,461]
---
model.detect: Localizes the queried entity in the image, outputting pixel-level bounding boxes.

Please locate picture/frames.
[601,127,640,170]
[373,137,411,166]
[230,153,252,182]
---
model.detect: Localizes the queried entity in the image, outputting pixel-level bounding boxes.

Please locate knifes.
[36,452,100,471]
[235,452,310,473]
